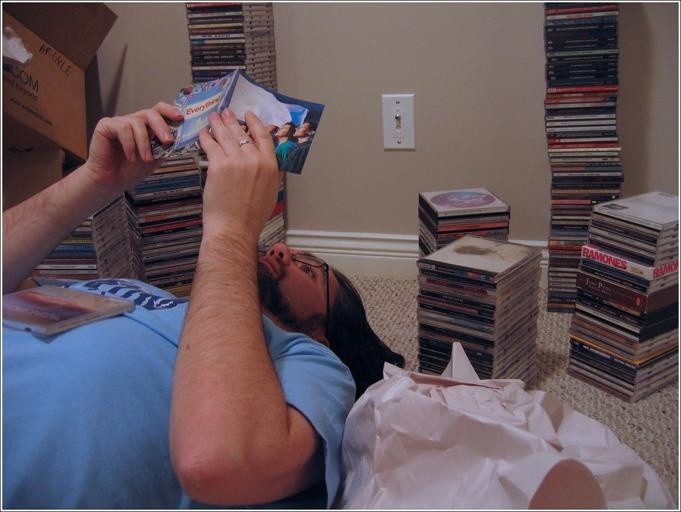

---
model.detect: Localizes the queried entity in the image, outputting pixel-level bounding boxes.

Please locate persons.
[274,121,297,171]
[2,102,405,508]
[279,120,317,176]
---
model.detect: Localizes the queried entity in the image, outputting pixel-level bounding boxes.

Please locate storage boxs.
[0,0,119,199]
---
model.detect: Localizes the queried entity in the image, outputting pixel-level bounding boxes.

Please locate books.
[2,283,137,342]
[147,68,327,177]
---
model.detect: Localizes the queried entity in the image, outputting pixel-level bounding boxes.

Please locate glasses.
[288,253,329,319]
